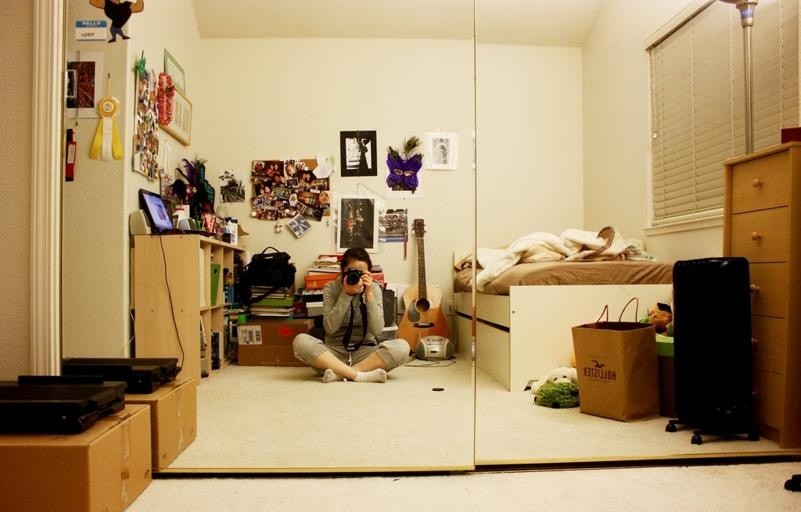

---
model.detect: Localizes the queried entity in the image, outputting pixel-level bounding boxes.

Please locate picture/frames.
[163,47,186,96]
[158,87,192,146]
[339,131,377,177]
[423,132,456,170]
[336,194,378,255]
[65,51,105,119]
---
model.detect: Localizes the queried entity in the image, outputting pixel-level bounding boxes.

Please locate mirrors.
[474,0,801,472]
[26,0,474,479]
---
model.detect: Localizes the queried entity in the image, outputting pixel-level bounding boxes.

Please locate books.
[250,283,294,319]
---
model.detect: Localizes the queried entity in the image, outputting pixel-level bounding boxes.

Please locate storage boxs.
[0,403,153,512]
[125,377,199,471]
[236,318,315,367]
[304,274,385,290]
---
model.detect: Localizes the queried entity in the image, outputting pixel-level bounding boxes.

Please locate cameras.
[342,268,363,285]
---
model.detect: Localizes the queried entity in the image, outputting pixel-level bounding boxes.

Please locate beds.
[453,247,674,392]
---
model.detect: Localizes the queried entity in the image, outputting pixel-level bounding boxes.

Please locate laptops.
[139,189,209,236]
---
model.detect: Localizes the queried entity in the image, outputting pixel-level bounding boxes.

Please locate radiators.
[665,256,760,445]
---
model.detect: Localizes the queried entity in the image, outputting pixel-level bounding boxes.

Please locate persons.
[357,139,370,170]
[342,208,372,248]
[293,248,411,384]
[250,161,330,235]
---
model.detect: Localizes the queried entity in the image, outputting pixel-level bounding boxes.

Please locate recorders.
[415,336,455,360]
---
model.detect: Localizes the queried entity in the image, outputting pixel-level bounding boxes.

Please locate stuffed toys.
[649,302,672,333]
[531,367,578,395]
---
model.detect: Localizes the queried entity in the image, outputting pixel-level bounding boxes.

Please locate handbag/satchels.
[237,245,298,306]
[571,296,661,422]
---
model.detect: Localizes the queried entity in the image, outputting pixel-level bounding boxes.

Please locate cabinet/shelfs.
[134,236,247,384]
[723,143,801,449]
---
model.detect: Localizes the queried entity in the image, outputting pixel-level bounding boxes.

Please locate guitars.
[396,219,452,353]
